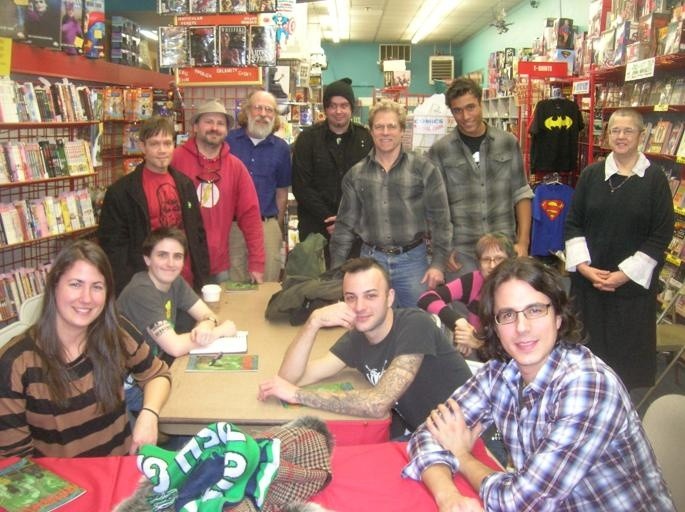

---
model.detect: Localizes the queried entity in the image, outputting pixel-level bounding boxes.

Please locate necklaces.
[603,173,637,195]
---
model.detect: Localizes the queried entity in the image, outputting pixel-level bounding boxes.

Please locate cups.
[200,284,222,313]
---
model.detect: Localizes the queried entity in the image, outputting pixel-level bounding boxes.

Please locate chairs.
[1,320,28,349]
[641,393,685,512]
[631,287,685,411]
[18,294,48,321]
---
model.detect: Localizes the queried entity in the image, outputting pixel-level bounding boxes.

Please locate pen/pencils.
[208,351,224,366]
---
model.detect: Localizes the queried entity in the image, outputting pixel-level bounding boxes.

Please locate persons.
[256,256,510,470]
[399,256,679,512]
[428,78,536,283]
[99,117,212,290]
[0,241,173,455]
[109,230,237,354]
[565,109,675,391]
[225,92,291,284]
[169,100,266,284]
[418,232,515,354]
[328,98,453,311]
[291,77,376,248]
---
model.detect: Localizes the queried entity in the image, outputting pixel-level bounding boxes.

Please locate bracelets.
[200,316,218,326]
[140,408,159,419]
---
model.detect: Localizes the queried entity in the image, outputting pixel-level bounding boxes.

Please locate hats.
[323,78,355,111]
[189,100,234,130]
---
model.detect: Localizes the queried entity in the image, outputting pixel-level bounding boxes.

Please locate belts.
[230,216,278,221]
[364,238,424,257]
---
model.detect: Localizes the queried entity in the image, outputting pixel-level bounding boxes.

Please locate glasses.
[252,104,273,113]
[492,301,553,325]
[196,171,221,183]
[478,256,505,264]
[373,122,398,130]
[609,126,638,135]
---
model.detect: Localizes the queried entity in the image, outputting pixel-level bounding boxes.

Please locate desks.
[158,280,391,447]
[0,435,510,511]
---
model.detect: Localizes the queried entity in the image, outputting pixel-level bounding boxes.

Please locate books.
[1,457,87,512]
[0,75,103,253]
[224,278,260,290]
[185,354,258,372]
[0,259,54,318]
[643,118,685,161]
[587,75,685,108]
[281,380,355,409]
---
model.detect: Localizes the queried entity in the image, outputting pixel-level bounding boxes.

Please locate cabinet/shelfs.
[458,43,517,141]
[158,0,329,250]
[0,0,180,322]
[517,0,684,377]
